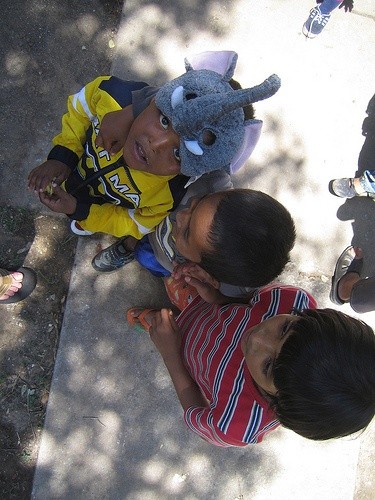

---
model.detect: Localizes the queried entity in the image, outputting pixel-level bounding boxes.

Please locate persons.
[26,51,281,239]
[127,263,375,447]
[92,169,296,288]
[333,167,375,314]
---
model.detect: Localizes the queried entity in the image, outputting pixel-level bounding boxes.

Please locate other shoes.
[332,177,359,198]
[67,219,96,236]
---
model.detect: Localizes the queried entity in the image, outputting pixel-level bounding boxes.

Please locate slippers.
[127,308,159,333]
[0,267,36,304]
[329,246,363,305]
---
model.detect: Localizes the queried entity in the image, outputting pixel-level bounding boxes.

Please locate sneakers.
[91,237,136,273]
[303,3,331,38]
